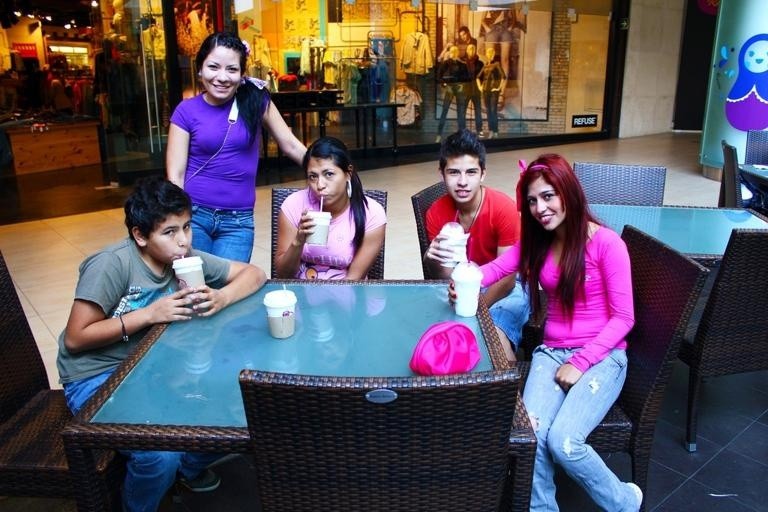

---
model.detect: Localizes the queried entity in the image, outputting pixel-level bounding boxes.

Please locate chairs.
[720,139,767,218]
[585,223,716,511]
[409,181,455,280]
[234,363,526,511]
[269,186,388,282]
[573,160,666,207]
[745,129,768,166]
[0,247,176,512]
[675,227,767,453]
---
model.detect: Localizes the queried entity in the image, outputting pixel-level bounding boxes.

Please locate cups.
[305,211,332,247]
[440,222,469,268]
[173,256,206,295]
[306,309,335,341]
[263,289,296,340]
[182,345,214,373]
[450,260,482,319]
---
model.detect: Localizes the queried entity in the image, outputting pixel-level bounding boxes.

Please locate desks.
[584,199,768,274]
[259,88,409,173]
[56,277,541,511]
[736,162,768,209]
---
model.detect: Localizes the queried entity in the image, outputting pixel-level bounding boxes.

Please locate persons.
[56,174,267,511]
[92,37,115,98]
[424,129,532,361]
[448,153,644,512]
[165,31,308,265]
[275,136,387,281]
[476,48,506,139]
[480,7,516,112]
[453,25,478,60]
[435,45,469,143]
[458,44,485,139]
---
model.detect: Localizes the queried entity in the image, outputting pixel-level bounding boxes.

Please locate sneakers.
[178,466,220,493]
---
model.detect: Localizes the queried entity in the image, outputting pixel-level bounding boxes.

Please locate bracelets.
[120,314,129,343]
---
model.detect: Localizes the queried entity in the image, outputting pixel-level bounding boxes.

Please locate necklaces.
[458,185,482,234]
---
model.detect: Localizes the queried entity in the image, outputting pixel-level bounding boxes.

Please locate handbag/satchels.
[409,321,481,375]
